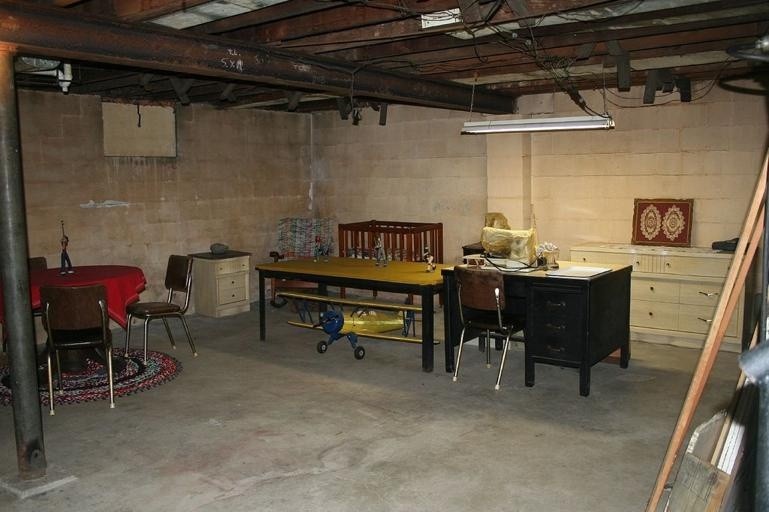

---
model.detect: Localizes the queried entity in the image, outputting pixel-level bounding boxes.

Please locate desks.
[0,265,147,346]
[441,261,633,396]
[255,256,456,372]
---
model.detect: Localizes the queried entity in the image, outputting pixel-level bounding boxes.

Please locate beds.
[338,219,443,305]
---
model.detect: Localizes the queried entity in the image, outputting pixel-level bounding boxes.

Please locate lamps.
[460,56,616,135]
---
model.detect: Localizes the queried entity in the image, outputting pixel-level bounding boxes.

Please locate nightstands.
[189,254,250,319]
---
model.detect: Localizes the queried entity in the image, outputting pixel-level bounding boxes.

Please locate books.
[545,266,613,280]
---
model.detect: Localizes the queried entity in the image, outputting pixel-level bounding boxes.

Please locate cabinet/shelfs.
[570,248,756,353]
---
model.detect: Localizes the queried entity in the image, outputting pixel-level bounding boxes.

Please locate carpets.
[0,347,183,407]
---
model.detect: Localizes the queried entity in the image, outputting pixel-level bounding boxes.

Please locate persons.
[424,247,436,272]
[373,238,387,267]
[59,220,74,274]
[314,235,328,263]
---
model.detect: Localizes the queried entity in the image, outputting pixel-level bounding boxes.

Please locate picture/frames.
[631,198,694,248]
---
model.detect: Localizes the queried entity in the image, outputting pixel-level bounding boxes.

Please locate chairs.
[452,265,522,391]
[30,257,47,271]
[269,218,334,308]
[125,255,198,365]
[40,285,116,416]
